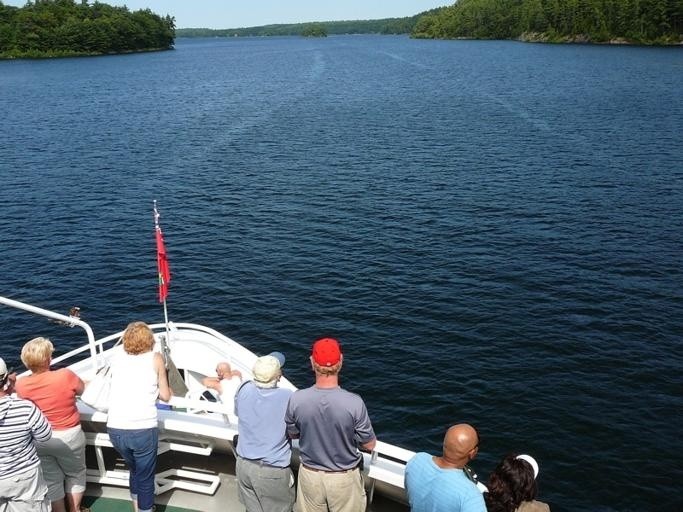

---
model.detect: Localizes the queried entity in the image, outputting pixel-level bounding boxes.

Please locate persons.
[15,336,92,511]
[286,338,376,512]
[403,422,487,512]
[482,454,549,511]
[199,362,240,403]
[106,320,172,511]
[0,354,52,512]
[234,352,293,512]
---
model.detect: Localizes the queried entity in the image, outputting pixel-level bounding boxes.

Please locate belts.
[302,463,357,474]
[244,459,287,468]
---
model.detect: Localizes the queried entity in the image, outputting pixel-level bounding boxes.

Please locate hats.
[0,357,8,383]
[253,351,285,383]
[514,454,538,480]
[311,338,339,367]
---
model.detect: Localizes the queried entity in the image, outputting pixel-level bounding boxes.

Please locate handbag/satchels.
[80,373,113,412]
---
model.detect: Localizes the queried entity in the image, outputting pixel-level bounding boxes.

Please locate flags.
[155,226,170,302]
